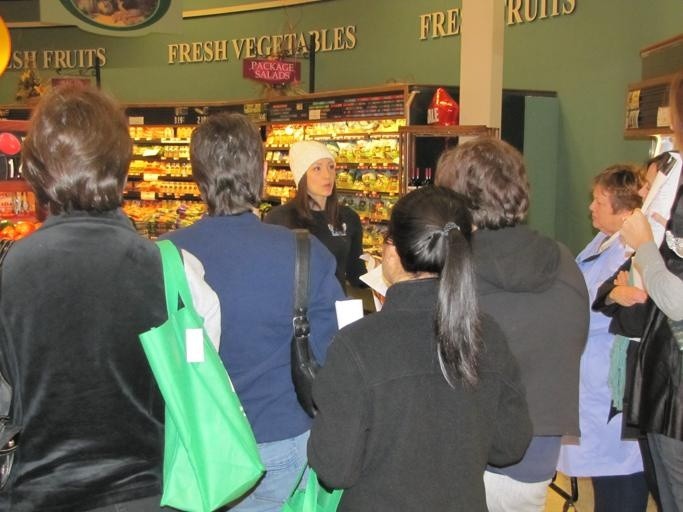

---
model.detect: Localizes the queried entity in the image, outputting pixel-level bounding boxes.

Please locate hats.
[288,140,336,193]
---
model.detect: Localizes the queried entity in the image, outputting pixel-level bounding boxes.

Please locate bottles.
[158,125,200,197]
[407,167,432,194]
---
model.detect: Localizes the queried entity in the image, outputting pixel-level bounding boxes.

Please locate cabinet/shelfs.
[120,85,523,225]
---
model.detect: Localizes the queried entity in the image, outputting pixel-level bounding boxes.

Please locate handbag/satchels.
[279,461,343,511]
[289,228,321,422]
[139,239,267,511]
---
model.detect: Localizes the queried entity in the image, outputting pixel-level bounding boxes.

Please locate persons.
[262,139,368,296]
[306,185,534,512]
[592,148,677,512]
[432,134,592,512]
[0,78,189,512]
[155,109,350,512]
[556,162,652,511]
[592,74,683,512]
